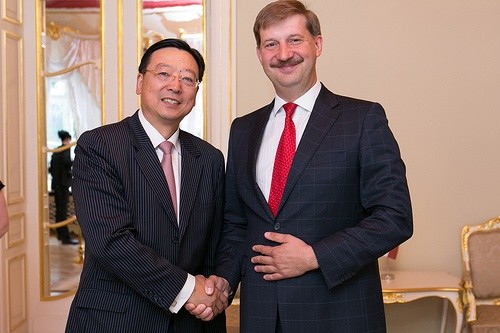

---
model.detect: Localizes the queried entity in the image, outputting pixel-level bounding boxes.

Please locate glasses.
[147,69,201,88]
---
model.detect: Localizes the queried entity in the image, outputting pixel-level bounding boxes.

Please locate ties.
[158,140,177,217]
[267,101,298,219]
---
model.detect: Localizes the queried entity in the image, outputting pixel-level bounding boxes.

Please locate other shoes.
[61,238,79,245]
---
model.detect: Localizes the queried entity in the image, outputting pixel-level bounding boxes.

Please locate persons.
[48,131,79,244]
[185,0,413,333]
[64,38,227,333]
[0,181,10,238]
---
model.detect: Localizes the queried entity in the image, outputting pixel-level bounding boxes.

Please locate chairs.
[461,217,500,333]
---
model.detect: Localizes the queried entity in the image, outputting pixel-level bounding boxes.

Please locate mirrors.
[35,0,105,303]
[137,0,207,140]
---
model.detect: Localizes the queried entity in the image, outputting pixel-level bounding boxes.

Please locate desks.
[379,270,465,333]
